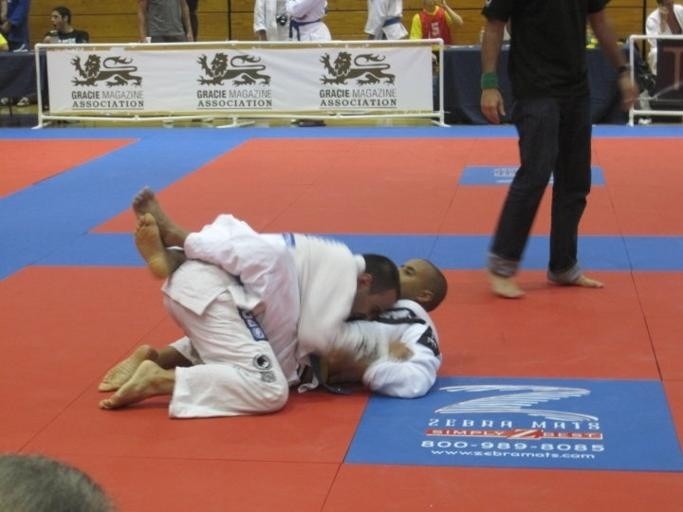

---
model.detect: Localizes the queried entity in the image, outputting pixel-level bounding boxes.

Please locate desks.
[442,44,637,127]
[0,52,53,119]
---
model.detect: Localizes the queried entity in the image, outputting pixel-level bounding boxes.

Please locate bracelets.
[614,64,627,78]
[480,71,499,90]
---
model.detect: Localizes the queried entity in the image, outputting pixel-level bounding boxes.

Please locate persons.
[97,232,413,420]
[42,7,89,125]
[644,0,683,79]
[410,0,464,47]
[179,0,198,42]
[136,0,214,125]
[253,0,296,41]
[0,33,10,51]
[129,184,448,399]
[284,0,331,129]
[1,449,116,512]
[472,1,640,298]
[0,0,31,107]
[364,0,408,41]
